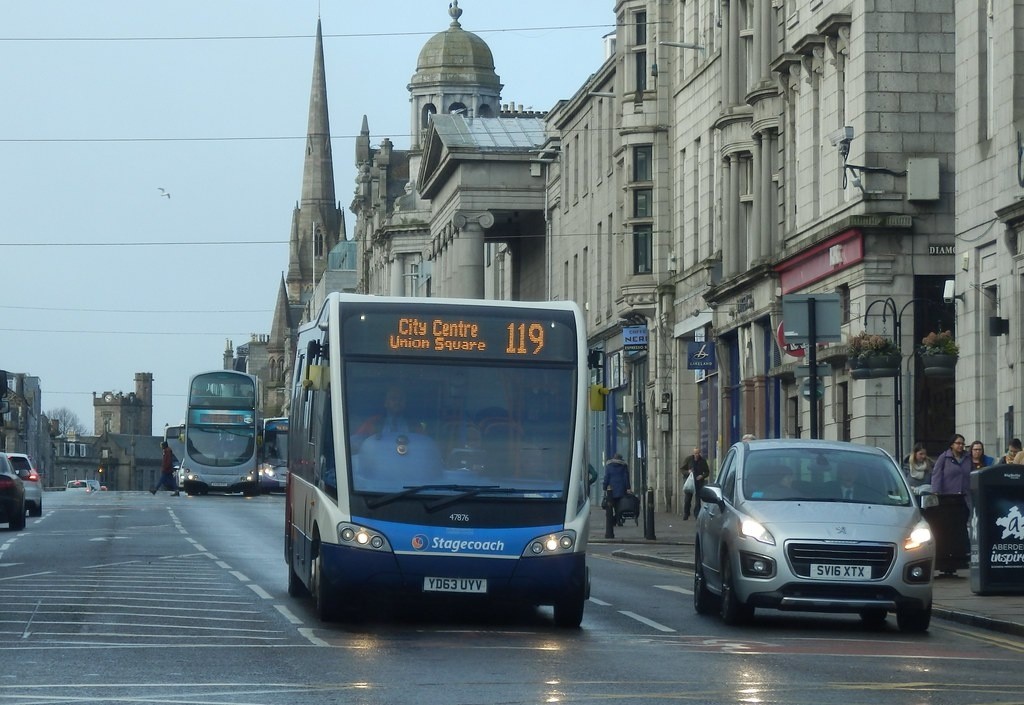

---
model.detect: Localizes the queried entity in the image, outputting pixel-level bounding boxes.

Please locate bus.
[161,425,186,489]
[180,369,264,497]
[285,292,620,618]
[257,418,290,493]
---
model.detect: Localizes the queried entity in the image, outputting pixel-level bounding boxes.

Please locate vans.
[66,480,104,492]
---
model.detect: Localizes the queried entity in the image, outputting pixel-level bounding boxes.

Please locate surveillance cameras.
[827,126,854,146]
[943,280,955,303]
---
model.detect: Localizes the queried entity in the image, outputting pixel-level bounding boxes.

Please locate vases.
[847,357,903,380]
[921,350,959,375]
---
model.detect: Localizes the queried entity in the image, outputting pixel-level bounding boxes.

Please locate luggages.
[618,492,639,526]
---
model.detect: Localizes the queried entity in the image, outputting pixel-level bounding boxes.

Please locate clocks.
[104,395,111,402]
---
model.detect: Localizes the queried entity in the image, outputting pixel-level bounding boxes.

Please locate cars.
[6,452,46,518]
[692,440,942,635]
[0,452,31,530]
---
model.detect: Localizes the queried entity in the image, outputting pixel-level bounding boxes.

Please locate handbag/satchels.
[171,454,178,468]
[601,496,607,509]
[682,472,696,493]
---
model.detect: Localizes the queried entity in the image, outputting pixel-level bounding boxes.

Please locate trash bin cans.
[970,464,1024,596]
[914,494,969,579]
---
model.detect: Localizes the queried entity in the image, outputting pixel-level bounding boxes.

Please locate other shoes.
[149,488,156,495]
[683,512,690,520]
[170,491,180,496]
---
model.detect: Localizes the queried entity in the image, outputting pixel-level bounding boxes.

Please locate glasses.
[953,441,965,446]
[972,448,982,451]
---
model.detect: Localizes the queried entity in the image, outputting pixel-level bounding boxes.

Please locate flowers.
[845,331,902,363]
[917,331,962,361]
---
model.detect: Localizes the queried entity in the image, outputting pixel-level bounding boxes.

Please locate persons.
[603,454,631,526]
[589,464,598,484]
[752,466,793,499]
[901,434,1024,569]
[827,460,868,499]
[680,446,709,520]
[149,442,180,497]
[742,434,755,441]
[356,388,428,434]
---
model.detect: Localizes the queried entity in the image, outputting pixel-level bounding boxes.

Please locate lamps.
[690,309,733,317]
[706,299,753,310]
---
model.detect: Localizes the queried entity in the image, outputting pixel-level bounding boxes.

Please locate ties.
[844,489,851,499]
[390,417,398,432]
